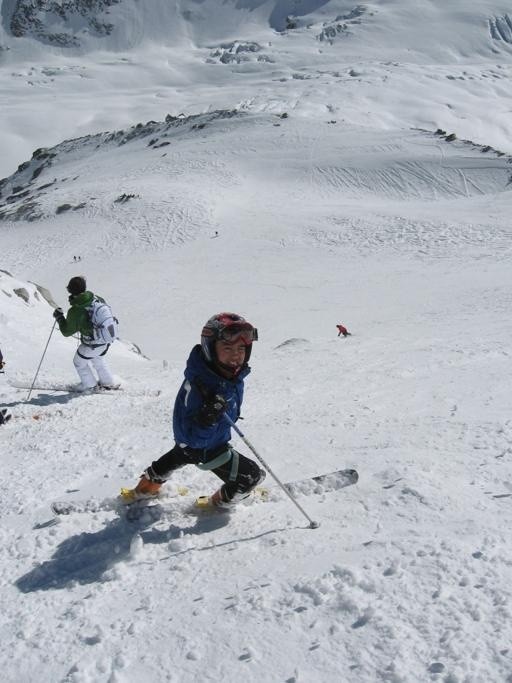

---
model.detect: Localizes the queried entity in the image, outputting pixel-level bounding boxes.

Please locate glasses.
[201,322,258,345]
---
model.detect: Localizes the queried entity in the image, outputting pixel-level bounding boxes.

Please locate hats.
[67,277,86,296]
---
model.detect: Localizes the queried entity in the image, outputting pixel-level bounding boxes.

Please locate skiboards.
[9,376,161,398]
[50,466,357,525]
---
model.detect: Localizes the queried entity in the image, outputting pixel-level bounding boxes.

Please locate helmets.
[201,313,253,380]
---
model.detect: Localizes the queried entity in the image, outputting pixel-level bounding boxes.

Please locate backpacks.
[83,295,119,344]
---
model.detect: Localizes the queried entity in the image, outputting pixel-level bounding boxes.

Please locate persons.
[336,325,352,338]
[135,313,261,509]
[53,277,117,392]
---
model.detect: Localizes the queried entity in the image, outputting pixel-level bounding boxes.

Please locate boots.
[134,467,167,499]
[209,484,262,510]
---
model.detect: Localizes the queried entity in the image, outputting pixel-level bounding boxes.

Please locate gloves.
[197,393,229,426]
[53,307,64,322]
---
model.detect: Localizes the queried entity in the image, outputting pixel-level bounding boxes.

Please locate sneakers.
[74,381,97,391]
[97,379,113,385]
